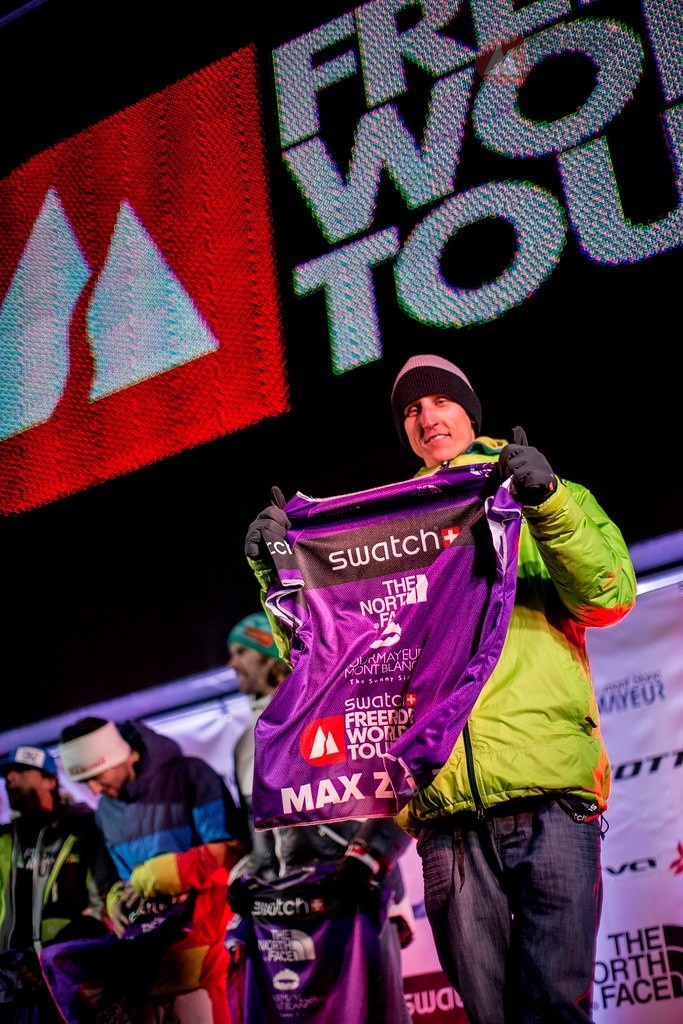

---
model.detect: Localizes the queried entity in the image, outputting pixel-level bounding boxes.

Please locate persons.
[50,716,236,1024]
[223,614,411,1024]
[0,743,124,1023]
[247,350,639,1024]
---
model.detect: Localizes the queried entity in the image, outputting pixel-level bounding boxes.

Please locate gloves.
[226,878,263,915]
[244,486,291,559]
[323,843,381,920]
[497,425,557,506]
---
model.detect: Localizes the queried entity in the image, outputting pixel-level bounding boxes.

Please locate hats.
[0,744,58,779]
[391,354,482,454]
[227,613,288,667]
[58,715,132,781]
